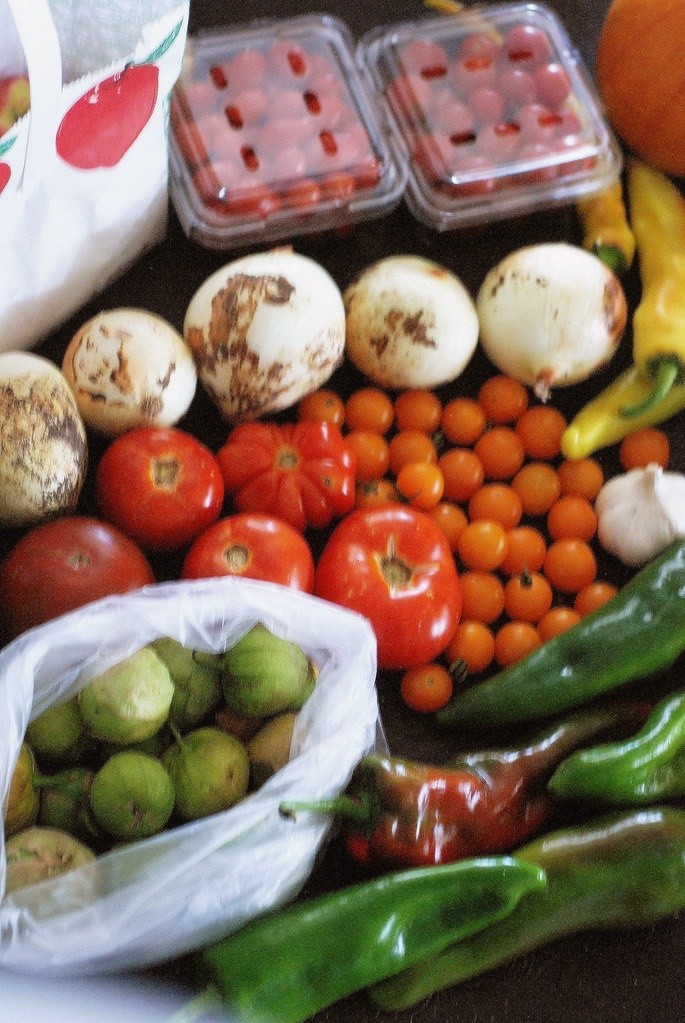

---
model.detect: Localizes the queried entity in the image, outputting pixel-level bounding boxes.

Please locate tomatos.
[167,22,599,242]
[0,388,665,711]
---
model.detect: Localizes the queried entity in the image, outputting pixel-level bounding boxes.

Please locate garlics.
[595,461,685,566]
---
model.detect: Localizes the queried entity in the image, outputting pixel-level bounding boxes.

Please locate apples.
[0,74,31,138]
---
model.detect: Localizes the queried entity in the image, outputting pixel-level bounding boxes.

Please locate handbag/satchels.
[0,0,189,350]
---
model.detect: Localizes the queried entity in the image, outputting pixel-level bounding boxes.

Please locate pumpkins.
[594,0,685,178]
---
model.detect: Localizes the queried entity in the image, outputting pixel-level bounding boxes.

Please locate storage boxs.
[175,15,413,245]
[357,3,623,233]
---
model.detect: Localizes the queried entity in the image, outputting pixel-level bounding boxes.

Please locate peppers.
[163,538,685,1023]
[560,139,684,459]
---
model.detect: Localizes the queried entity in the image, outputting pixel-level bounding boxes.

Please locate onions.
[0,243,626,524]
